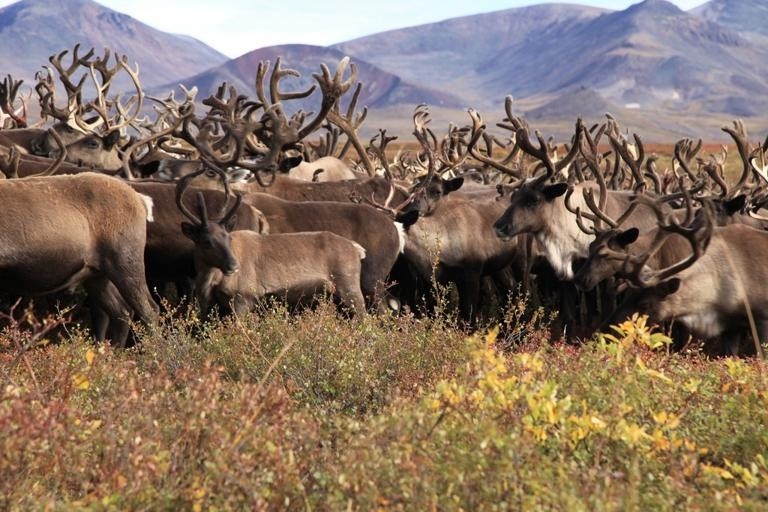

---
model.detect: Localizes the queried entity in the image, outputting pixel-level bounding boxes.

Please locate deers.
[1,41,767,357]
